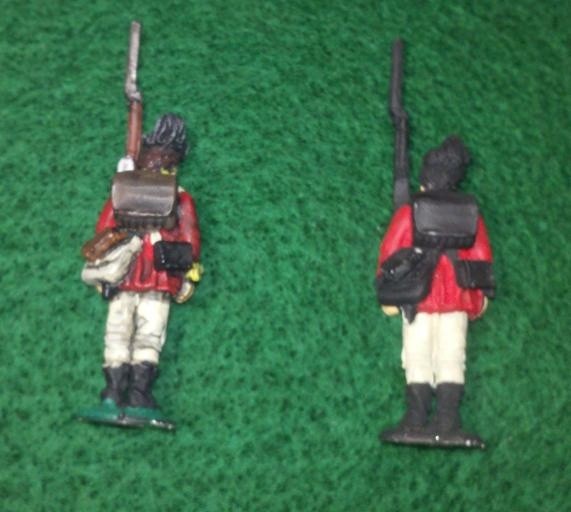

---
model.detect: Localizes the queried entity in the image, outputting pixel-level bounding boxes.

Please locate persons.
[79,114,205,431]
[376,133,497,449]
